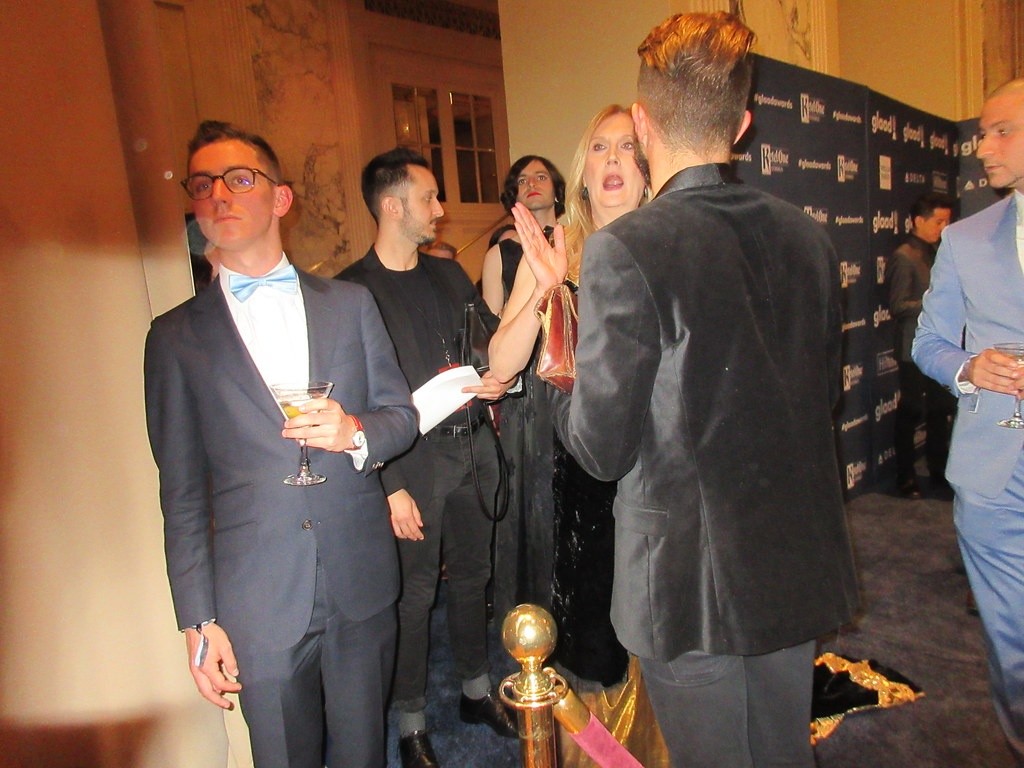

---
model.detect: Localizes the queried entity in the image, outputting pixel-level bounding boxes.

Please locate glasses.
[179,167,279,201]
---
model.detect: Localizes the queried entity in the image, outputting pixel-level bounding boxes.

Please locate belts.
[430,414,486,438]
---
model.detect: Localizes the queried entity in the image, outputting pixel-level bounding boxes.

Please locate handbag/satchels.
[459,303,493,373]
[532,284,579,395]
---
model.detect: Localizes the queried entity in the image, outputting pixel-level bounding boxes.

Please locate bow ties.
[229,265,299,304]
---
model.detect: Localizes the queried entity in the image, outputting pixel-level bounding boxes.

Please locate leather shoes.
[396,731,438,767]
[897,474,922,498]
[930,470,947,483]
[459,686,518,739]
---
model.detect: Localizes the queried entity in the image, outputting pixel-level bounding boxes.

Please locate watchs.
[344,415,366,450]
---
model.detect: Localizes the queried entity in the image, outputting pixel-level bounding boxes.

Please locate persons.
[333,147,526,768]
[542,9,865,768]
[911,77,1024,755]
[481,154,568,664]
[488,104,653,693]
[142,119,421,768]
[884,195,955,502]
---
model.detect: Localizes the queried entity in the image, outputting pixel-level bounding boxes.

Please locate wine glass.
[271,380,334,487]
[992,343,1024,430]
